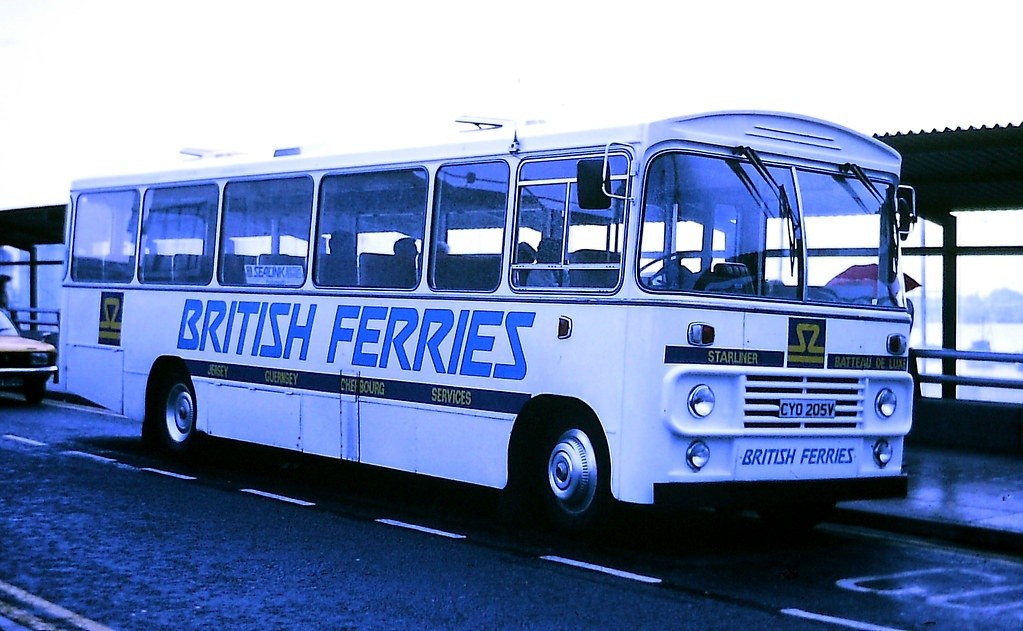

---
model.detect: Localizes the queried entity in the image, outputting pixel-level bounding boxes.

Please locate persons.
[323,230,358,287]
[436,241,449,255]
[525,238,571,287]
[384,238,419,289]
[906,298,925,428]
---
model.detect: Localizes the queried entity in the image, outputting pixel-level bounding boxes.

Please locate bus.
[57,113,914,550]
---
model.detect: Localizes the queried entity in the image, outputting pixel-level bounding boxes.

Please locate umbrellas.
[821,263,924,296]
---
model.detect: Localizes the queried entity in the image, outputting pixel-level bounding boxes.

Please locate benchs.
[77,242,755,294]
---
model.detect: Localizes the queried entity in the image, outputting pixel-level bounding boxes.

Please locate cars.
[0,310,58,404]
